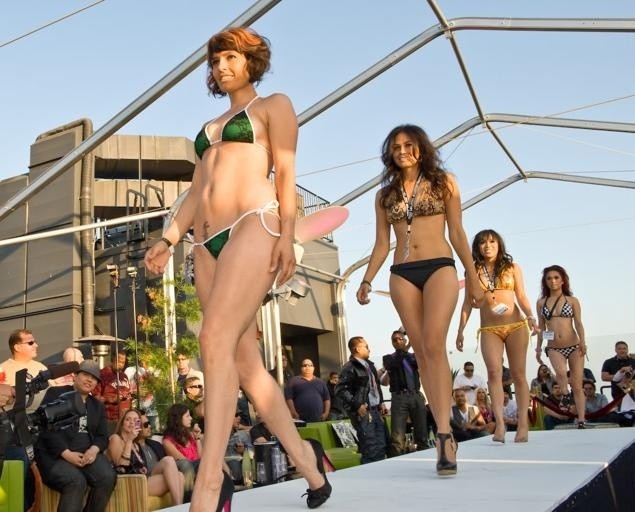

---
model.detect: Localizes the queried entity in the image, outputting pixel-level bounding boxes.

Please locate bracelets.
[160,237,175,256]
[525,315,536,321]
[360,281,372,293]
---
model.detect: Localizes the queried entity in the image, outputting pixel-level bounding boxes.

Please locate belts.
[397,388,418,395]
[366,404,381,412]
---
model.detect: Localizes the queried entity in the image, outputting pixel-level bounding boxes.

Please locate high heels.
[305,437,337,508]
[213,467,236,512]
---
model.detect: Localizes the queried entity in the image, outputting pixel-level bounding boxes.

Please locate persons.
[456,229,538,444]
[535,265,588,429]
[142,26,336,512]
[449,341,635,442]
[2,331,436,512]
[356,124,485,475]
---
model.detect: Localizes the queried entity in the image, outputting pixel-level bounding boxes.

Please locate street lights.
[125,264,141,409]
[105,262,121,424]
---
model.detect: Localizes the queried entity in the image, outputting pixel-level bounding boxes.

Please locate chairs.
[0,384,544,512]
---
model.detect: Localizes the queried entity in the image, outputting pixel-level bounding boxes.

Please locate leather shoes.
[492,429,506,443]
[514,432,528,442]
[559,394,571,410]
[436,430,459,475]
[576,420,587,429]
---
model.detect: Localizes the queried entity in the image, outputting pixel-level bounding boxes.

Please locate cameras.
[626,371,632,378]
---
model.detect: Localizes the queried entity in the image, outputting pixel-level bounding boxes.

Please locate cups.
[243,445,288,491]
[410,443,417,451]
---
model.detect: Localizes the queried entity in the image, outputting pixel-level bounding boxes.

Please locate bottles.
[404,432,412,453]
[241,443,252,479]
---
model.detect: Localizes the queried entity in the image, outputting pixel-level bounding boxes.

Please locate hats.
[73,360,104,384]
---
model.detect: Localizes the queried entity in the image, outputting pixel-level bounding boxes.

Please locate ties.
[364,361,377,398]
[403,358,413,375]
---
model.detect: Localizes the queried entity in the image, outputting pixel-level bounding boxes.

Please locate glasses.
[16,340,34,345]
[299,364,315,368]
[464,368,474,372]
[184,385,202,389]
[143,420,151,428]
